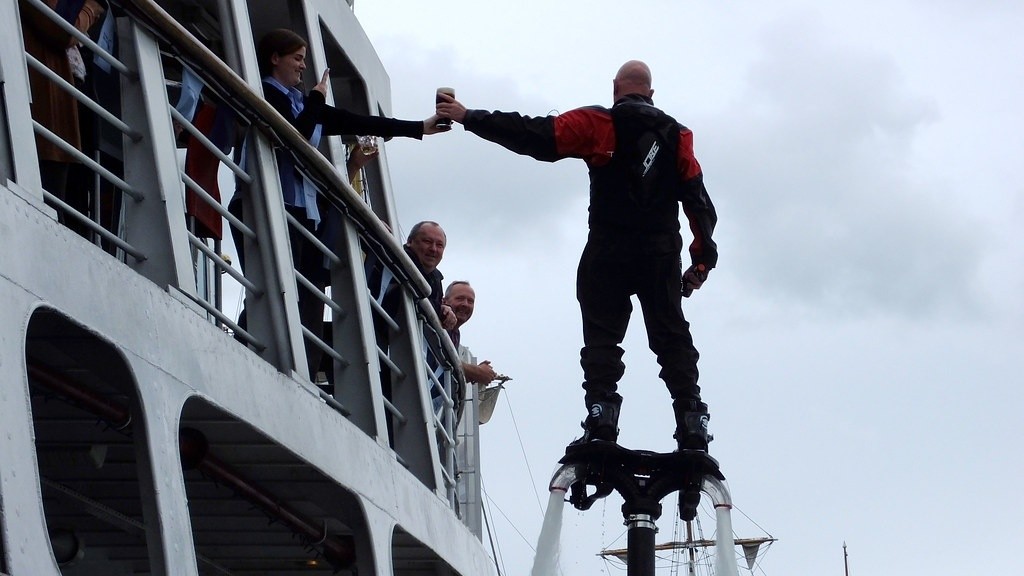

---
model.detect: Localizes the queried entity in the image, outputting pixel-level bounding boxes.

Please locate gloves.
[683,264,709,297]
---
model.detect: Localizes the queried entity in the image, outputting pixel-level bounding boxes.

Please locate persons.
[229,26,454,382]
[436,59,718,454]
[15,0,123,265]
[376,217,498,467]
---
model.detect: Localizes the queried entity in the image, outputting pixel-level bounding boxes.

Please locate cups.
[436,87,455,128]
[357,135,375,155]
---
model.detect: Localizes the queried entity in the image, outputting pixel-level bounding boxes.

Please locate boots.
[569,393,623,445]
[673,400,713,453]
